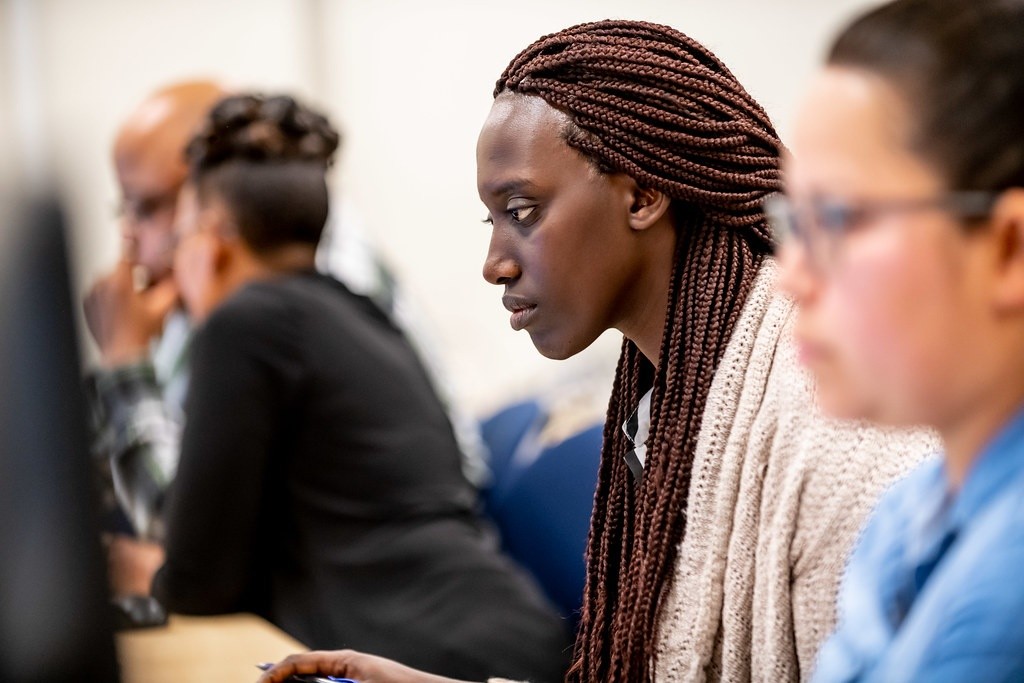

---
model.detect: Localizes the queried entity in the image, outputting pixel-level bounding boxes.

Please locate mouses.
[112,591,168,629]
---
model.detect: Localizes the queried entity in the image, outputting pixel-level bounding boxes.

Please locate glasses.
[762,193,994,244]
[117,185,181,220]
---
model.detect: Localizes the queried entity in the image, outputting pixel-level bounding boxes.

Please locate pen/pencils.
[253,661,359,683]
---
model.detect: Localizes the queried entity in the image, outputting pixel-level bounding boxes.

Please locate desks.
[111,611,311,682]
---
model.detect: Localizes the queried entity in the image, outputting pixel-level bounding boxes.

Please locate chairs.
[482,398,547,513]
[488,423,605,632]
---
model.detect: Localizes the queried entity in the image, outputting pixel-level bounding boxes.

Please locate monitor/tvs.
[0,182,125,683]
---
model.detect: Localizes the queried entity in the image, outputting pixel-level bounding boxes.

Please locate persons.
[94,90,578,680]
[780,0,1024,682]
[80,78,409,549]
[260,15,941,682]
